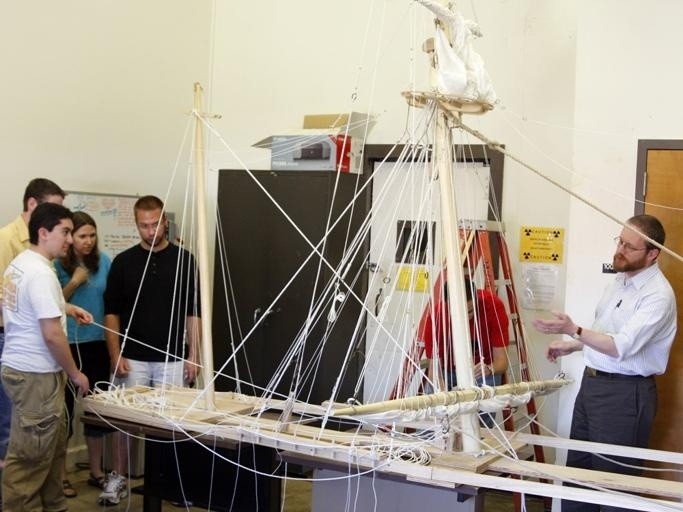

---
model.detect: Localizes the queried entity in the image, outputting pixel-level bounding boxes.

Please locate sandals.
[87,472,108,489]
[63,480,77,497]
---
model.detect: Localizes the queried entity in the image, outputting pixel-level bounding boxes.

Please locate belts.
[585,365,621,378]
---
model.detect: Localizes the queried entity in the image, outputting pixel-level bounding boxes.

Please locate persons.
[422,272,513,439]
[528,212,677,512]
[48,209,112,500]
[0,200,96,512]
[0,176,67,470]
[96,193,203,506]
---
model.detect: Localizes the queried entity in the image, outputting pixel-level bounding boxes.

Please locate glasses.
[615,236,647,253]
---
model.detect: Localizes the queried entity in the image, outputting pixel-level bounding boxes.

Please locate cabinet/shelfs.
[213,169,367,405]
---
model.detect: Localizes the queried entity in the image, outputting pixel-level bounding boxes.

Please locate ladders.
[375,217,552,512]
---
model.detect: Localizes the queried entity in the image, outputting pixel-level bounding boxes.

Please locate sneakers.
[98,470,128,505]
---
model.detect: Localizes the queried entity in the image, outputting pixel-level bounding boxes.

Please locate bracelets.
[487,365,494,377]
[573,324,584,339]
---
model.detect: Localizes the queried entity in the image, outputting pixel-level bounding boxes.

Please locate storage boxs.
[251,112,369,171]
